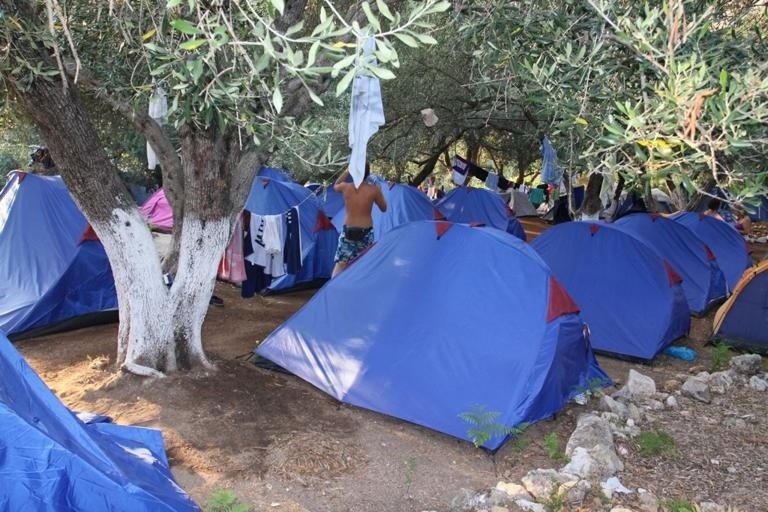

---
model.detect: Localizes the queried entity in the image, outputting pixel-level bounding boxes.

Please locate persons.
[702,198,726,222]
[728,200,754,257]
[331,162,387,280]
[426,176,438,200]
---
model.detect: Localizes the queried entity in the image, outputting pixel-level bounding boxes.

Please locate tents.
[665,210,754,297]
[251,218,622,460]
[437,185,528,244]
[1,168,175,341]
[0,330,205,512]
[137,185,179,234]
[610,211,733,319]
[703,257,767,357]
[529,220,693,366]
[317,174,390,223]
[217,165,344,297]
[329,176,446,253]
